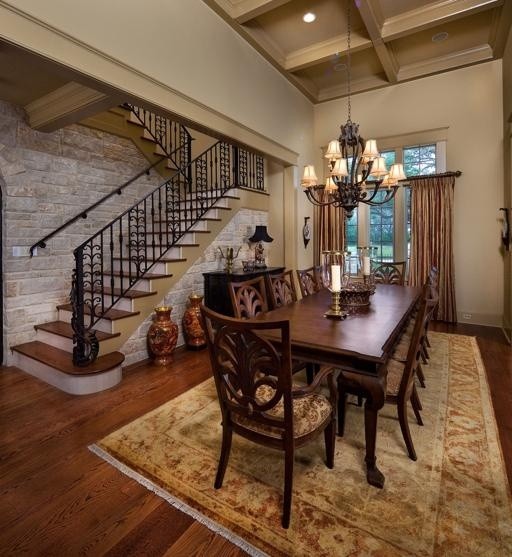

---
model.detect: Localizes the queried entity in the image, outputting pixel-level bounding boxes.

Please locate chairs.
[225,263,332,318]
[333,257,436,467]
[200,308,339,529]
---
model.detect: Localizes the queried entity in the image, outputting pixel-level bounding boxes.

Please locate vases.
[182,295,209,351]
[147,307,178,367]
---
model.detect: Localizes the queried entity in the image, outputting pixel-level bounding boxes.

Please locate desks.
[233,281,421,488]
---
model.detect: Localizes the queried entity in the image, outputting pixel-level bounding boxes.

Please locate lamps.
[248,226,274,269]
[300,8,407,221]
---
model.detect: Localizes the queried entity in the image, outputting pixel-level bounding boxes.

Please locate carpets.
[87,331,512,557]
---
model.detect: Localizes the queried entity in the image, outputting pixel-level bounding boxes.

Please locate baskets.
[340,282,376,306]
